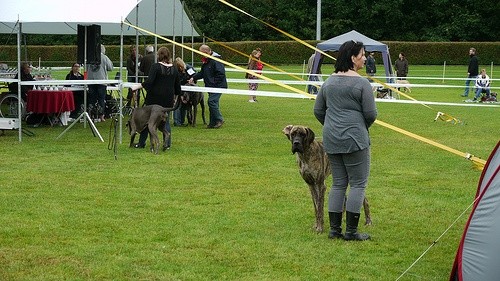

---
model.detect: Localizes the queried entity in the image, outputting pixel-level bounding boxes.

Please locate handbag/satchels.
[257,65,262,74]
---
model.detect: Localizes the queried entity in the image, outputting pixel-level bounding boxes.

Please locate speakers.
[77,24,101,64]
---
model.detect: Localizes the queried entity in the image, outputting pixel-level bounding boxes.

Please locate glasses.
[72,65,80,67]
[351,39,358,50]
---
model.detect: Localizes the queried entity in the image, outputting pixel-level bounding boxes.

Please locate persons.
[87,44,113,121]
[187,44,227,128]
[126,44,145,110]
[366,51,376,76]
[173,56,197,127]
[11,62,34,102]
[139,44,155,81]
[314,40,377,240]
[395,52,409,91]
[134,47,181,150]
[64,62,84,119]
[473,68,490,101]
[460,47,479,96]
[244,48,264,102]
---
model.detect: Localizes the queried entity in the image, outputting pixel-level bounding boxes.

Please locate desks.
[27,86,120,128]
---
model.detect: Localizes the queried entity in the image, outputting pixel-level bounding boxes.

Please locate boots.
[344,209,370,240]
[328,211,344,239]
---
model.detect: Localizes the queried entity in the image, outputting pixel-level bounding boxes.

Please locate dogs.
[124,96,180,155]
[491,92,497,101]
[393,80,411,94]
[282,125,372,234]
[179,83,205,127]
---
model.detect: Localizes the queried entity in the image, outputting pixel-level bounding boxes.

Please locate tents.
[448,139,500,281]
[307,30,394,97]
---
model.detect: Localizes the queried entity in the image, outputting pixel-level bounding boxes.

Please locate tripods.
[57,26,106,142]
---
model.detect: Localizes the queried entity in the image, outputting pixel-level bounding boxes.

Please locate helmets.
[146,45,154,53]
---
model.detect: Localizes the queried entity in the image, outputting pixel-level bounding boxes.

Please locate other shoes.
[174,121,184,125]
[133,142,146,147]
[124,104,131,107]
[473,97,477,102]
[206,124,215,127]
[248,98,259,102]
[100,114,106,121]
[214,119,225,127]
